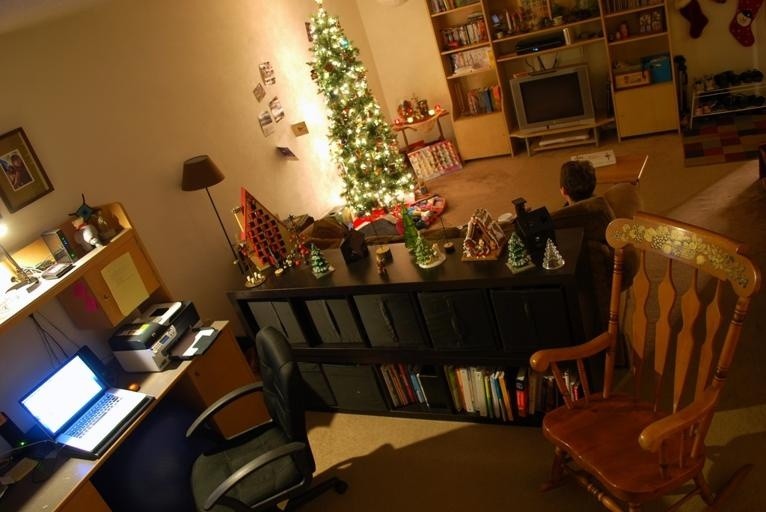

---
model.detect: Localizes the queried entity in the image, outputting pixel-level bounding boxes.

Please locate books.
[602,0,664,14]
[432,0,501,115]
[380,362,581,421]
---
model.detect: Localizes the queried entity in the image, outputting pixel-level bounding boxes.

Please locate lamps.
[180,154,247,279]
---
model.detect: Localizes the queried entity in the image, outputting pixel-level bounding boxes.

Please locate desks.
[3,199,266,512]
[391,109,450,152]
[595,153,648,207]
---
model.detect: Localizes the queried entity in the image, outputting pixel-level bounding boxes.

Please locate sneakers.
[692,69,764,116]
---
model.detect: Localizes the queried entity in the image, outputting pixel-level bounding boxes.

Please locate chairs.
[546,185,650,367]
[185,323,348,512]
[527,210,760,512]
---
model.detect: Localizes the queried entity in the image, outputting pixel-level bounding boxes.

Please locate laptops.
[18,352,147,453]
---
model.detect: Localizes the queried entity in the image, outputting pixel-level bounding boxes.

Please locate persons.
[560,159,597,206]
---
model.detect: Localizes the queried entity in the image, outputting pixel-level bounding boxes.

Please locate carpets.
[680,114,766,168]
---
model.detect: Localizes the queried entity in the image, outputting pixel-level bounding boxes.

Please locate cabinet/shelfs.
[425,0,680,162]
[226,227,606,426]
[690,79,766,129]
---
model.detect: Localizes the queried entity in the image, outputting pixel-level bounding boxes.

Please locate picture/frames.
[0,126,54,215]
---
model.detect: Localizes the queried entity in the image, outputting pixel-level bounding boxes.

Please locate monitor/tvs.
[510,64,595,133]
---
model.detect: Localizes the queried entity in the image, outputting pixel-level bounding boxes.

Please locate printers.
[108,300,220,372]
